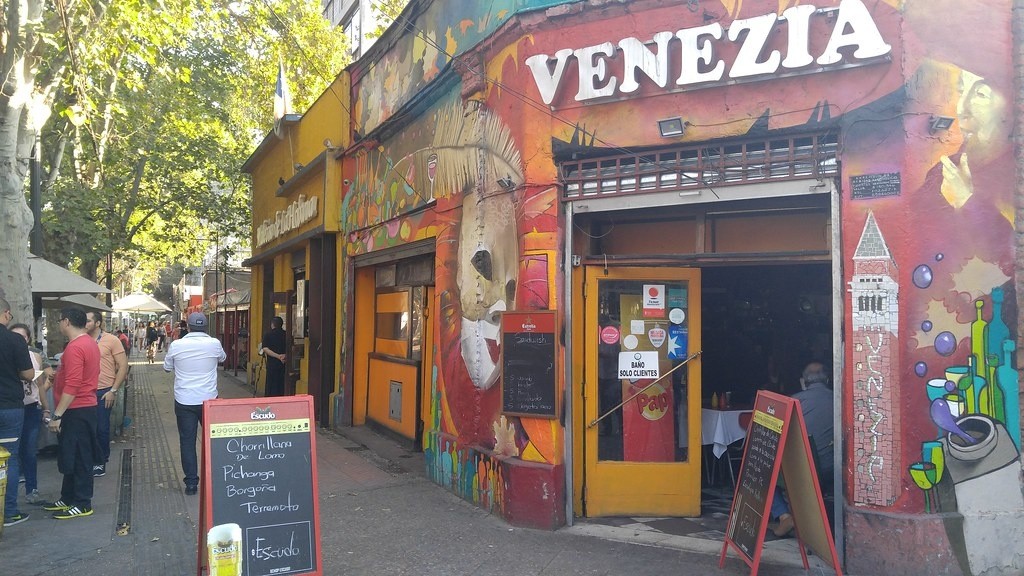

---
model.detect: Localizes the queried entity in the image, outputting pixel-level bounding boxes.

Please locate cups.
[207,527,242,576]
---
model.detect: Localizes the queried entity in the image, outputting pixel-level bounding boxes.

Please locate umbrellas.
[25,254,114,313]
[112,292,172,355]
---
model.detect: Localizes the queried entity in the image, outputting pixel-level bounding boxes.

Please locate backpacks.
[149,326,158,342]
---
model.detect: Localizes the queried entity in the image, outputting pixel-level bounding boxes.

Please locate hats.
[188,312,208,327]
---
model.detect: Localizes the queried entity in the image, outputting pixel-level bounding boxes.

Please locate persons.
[42,308,100,518]
[86,311,128,476]
[115,319,187,365]
[263,317,286,396]
[0,298,35,526]
[164,312,227,495]
[770,363,834,536]
[9,324,53,504]
[600,321,622,436]
[54,342,68,359]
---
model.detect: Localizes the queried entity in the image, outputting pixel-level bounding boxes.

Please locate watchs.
[52,413,62,421]
[111,389,117,395]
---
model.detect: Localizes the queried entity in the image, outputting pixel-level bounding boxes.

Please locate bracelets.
[42,409,51,413]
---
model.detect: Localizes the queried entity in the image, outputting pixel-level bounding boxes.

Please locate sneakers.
[43,499,69,511]
[52,503,94,518]
[3,510,30,526]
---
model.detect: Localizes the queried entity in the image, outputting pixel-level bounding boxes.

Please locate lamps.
[496,177,510,188]
[656,116,685,139]
[930,115,955,130]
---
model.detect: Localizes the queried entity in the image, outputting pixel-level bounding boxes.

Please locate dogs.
[145,344,156,365]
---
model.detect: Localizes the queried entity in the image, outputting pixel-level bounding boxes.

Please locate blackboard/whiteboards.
[501,309,558,419]
[198,394,322,576]
[725,390,840,569]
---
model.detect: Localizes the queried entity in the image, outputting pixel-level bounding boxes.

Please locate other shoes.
[18,474,26,483]
[25,488,46,505]
[185,485,197,495]
[774,516,796,536]
[93,464,105,477]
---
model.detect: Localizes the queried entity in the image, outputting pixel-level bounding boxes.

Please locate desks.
[678,401,753,488]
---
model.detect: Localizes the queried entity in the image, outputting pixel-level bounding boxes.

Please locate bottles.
[720,393,727,411]
[726,391,732,411]
[712,392,718,410]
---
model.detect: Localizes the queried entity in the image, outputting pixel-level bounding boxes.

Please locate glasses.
[5,311,14,321]
[55,318,70,325]
